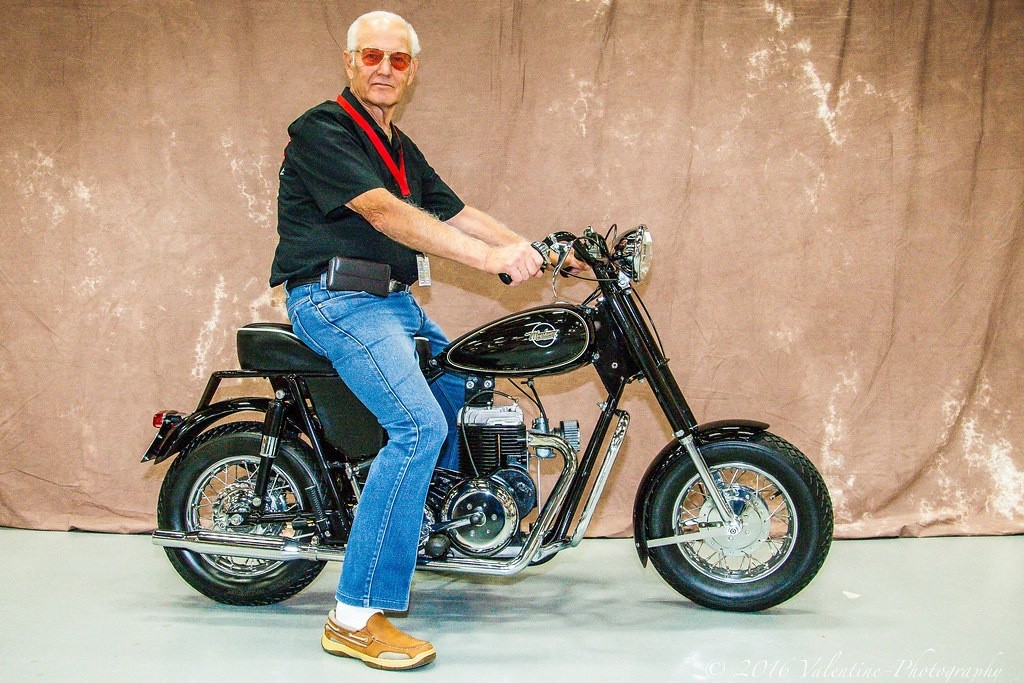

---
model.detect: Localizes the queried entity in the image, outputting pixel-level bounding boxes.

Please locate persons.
[269,11,591,670]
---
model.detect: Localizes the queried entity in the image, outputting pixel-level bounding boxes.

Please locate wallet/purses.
[327,255,391,298]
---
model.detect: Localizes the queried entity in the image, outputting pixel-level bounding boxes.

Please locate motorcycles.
[141,223,833,614]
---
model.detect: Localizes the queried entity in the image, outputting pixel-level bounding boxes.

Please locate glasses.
[350,47,411,71]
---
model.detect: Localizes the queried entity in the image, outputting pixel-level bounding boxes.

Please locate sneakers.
[321,610,436,670]
